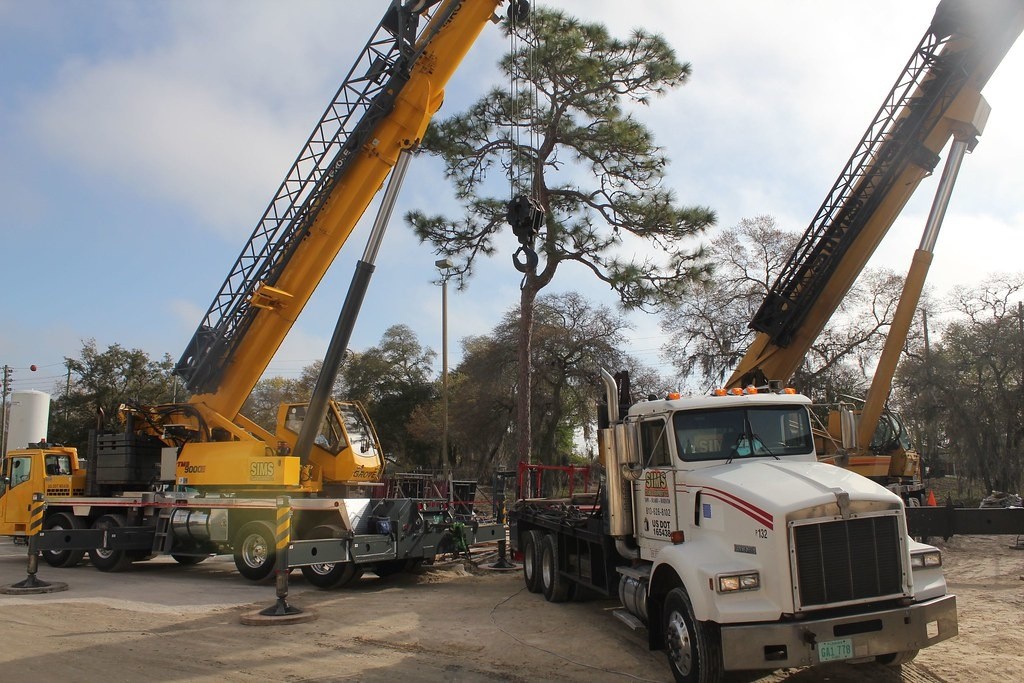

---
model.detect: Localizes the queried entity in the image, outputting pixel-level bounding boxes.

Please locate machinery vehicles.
[597,0,1024,507]
[0,1,546,590]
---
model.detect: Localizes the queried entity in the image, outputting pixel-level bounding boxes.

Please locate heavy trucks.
[505,366,959,683]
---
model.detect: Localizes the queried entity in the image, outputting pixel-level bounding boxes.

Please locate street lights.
[434,259,454,472]
